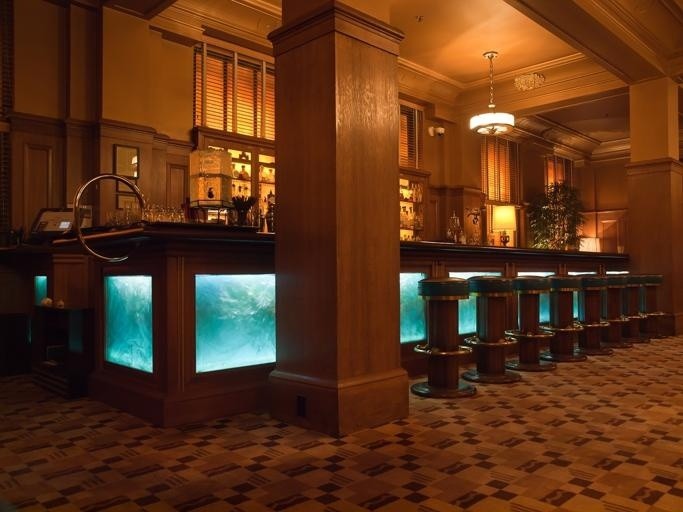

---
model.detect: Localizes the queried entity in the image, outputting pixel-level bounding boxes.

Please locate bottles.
[398,181,424,241]
[231,162,275,204]
[265,205,274,232]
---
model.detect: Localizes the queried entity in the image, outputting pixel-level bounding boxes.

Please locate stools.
[410,273,668,399]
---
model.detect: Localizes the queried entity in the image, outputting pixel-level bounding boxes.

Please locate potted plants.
[231,196,258,225]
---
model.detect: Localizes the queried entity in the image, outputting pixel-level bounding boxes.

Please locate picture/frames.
[113,143,139,210]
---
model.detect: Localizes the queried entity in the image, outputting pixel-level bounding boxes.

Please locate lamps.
[491,205,517,247]
[189,148,233,225]
[468,51,515,137]
[577,236,602,253]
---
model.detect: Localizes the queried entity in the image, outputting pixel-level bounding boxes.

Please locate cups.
[616,245,625,254]
[141,202,185,224]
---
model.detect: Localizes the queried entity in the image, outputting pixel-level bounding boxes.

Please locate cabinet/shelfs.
[28,302,96,399]
[399,167,432,241]
[192,125,275,231]
